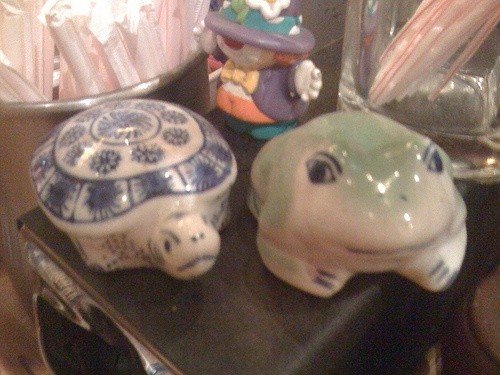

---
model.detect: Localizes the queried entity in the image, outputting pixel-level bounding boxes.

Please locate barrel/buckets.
[1,50,215,324]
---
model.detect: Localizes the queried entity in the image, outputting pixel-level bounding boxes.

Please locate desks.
[16,28,500,375]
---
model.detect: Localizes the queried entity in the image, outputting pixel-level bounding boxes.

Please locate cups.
[338,2,500,188]
